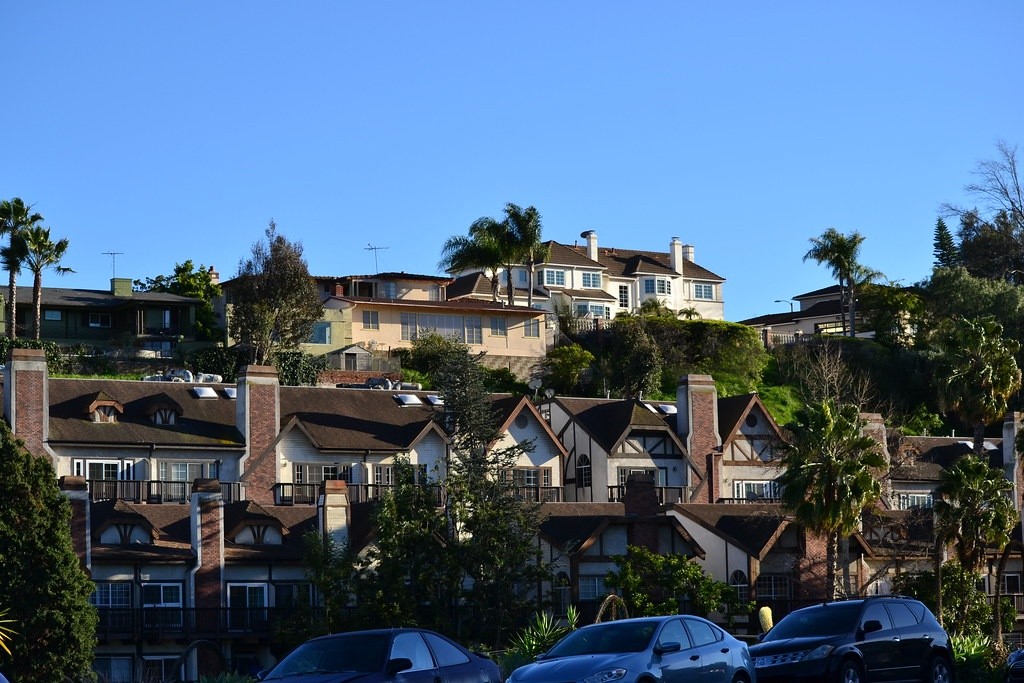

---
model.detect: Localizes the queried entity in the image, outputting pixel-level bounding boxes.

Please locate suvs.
[749,592,956,683]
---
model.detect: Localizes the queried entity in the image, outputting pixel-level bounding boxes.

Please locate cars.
[257,628,501,683]
[502,612,755,683]
